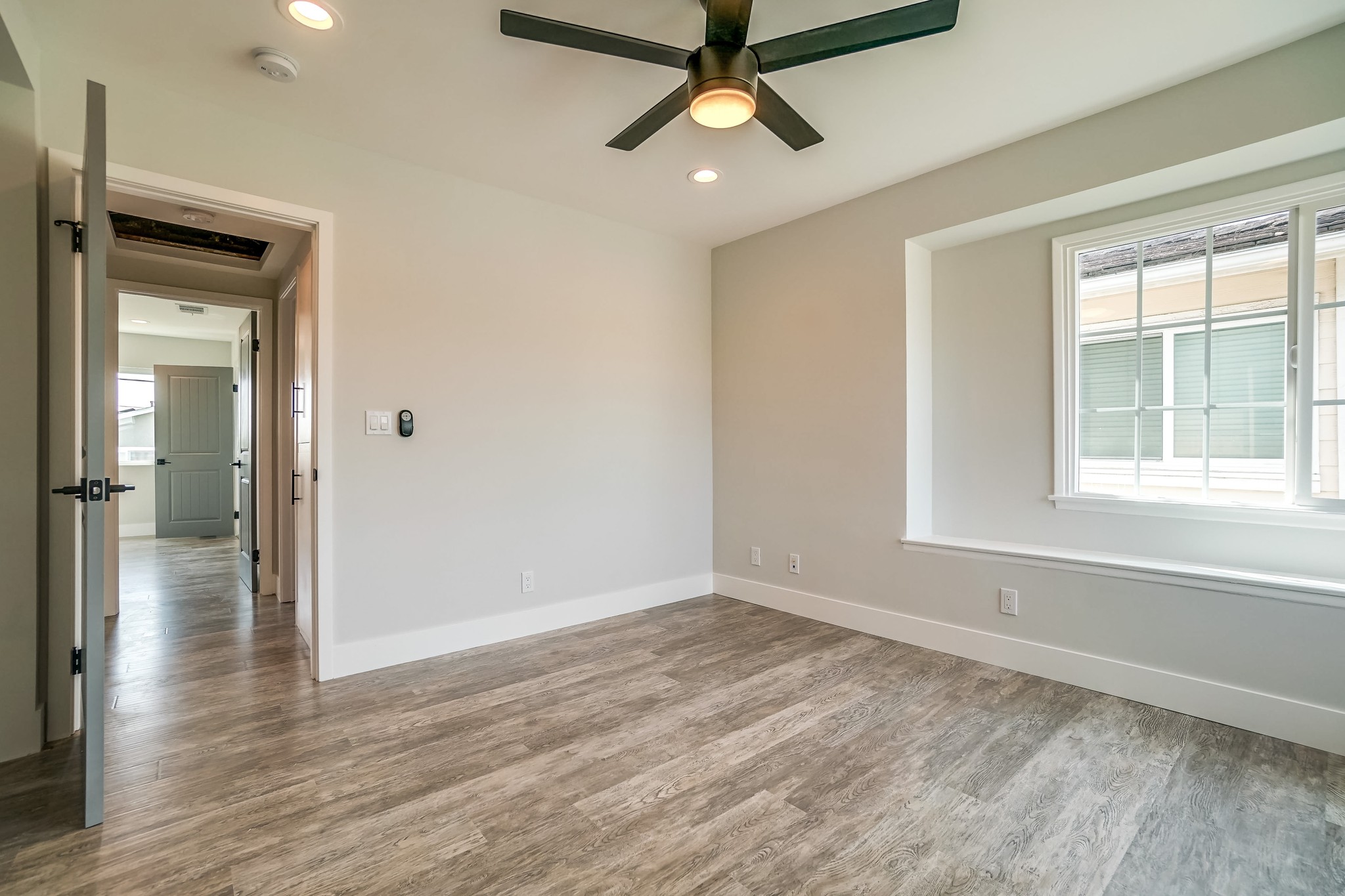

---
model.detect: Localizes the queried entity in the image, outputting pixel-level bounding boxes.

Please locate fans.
[500,0,959,151]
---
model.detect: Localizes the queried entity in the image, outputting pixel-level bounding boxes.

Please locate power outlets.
[751,548,760,566]
[1000,588,1017,616]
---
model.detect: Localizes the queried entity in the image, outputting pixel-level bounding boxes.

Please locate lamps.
[687,43,758,128]
